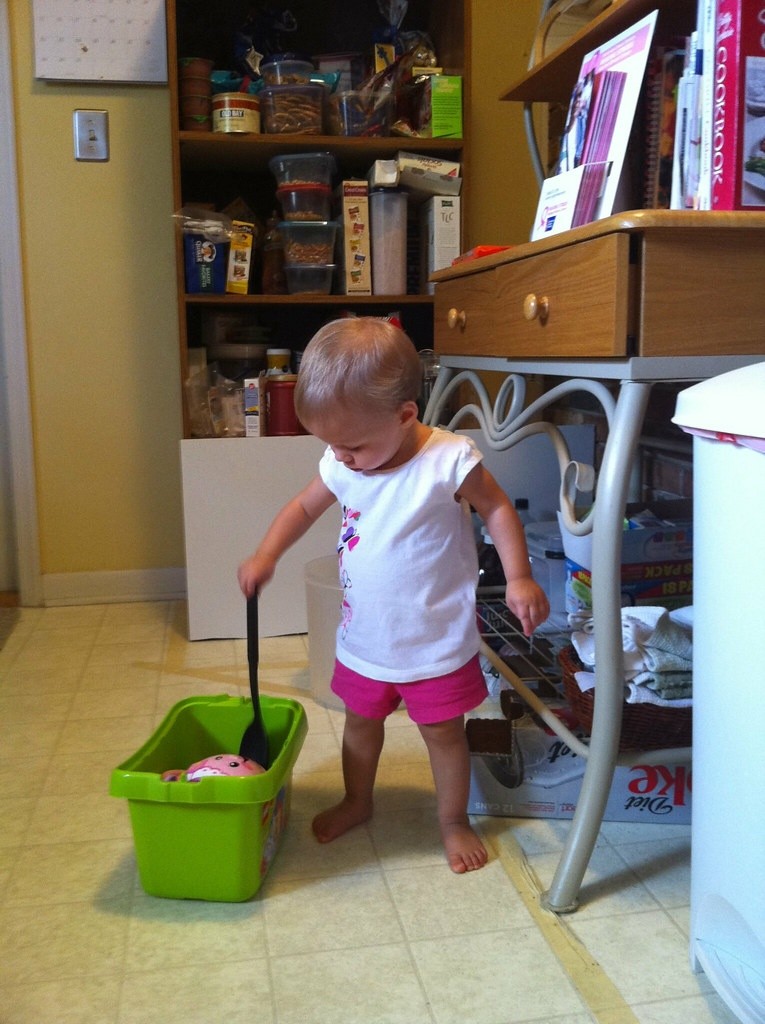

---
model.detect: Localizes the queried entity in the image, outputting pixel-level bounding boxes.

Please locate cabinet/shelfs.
[162,1,601,646]
[420,1,765,916]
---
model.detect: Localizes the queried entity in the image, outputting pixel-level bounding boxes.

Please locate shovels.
[235,583,271,769]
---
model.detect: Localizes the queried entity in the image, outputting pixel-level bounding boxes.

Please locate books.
[531,0,764,246]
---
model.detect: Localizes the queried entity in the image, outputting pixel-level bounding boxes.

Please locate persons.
[558,80,588,175]
[237,320,551,876]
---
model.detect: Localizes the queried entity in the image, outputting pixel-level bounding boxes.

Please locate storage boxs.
[108,693,310,907]
[460,687,692,829]
